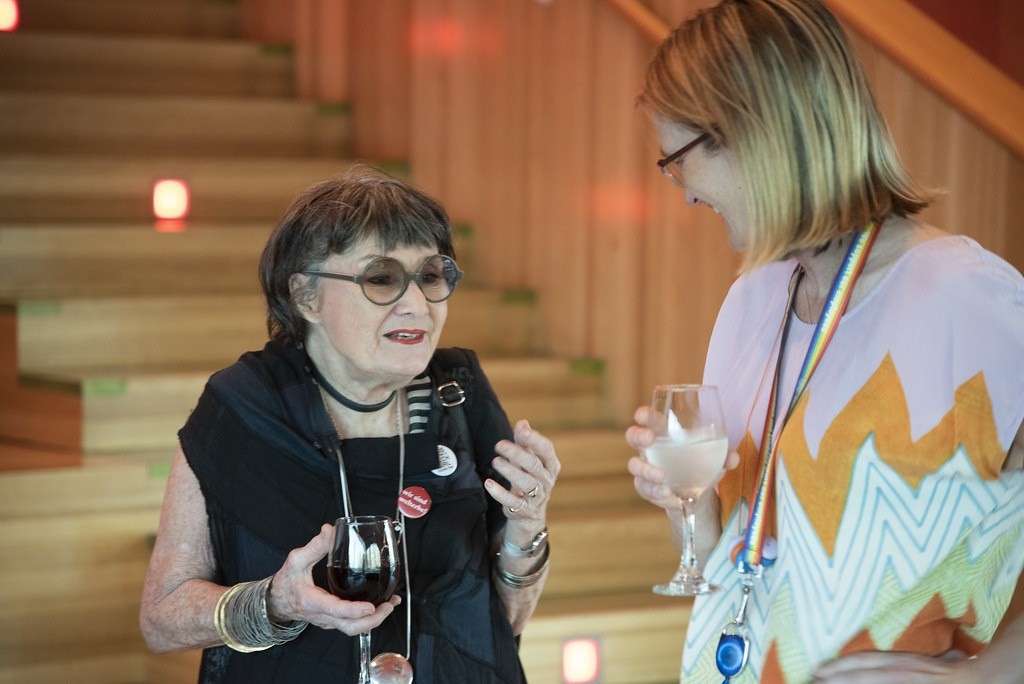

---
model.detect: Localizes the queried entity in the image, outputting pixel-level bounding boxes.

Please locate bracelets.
[493,548,551,589]
[213,575,309,654]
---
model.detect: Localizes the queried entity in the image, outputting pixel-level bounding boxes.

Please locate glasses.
[308,256,462,305]
[657,131,724,189]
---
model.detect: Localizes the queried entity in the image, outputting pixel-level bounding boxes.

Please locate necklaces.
[303,345,418,683]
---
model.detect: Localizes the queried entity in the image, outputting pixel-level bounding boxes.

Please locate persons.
[139,161,563,684]
[626,1,1024,684]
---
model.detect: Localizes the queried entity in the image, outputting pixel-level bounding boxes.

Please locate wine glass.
[645,381,729,598]
[327,515,400,684]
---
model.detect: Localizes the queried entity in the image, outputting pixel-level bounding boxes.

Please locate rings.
[509,497,525,513]
[518,482,541,497]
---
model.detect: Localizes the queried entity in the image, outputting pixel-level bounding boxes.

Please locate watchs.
[500,526,550,558]
